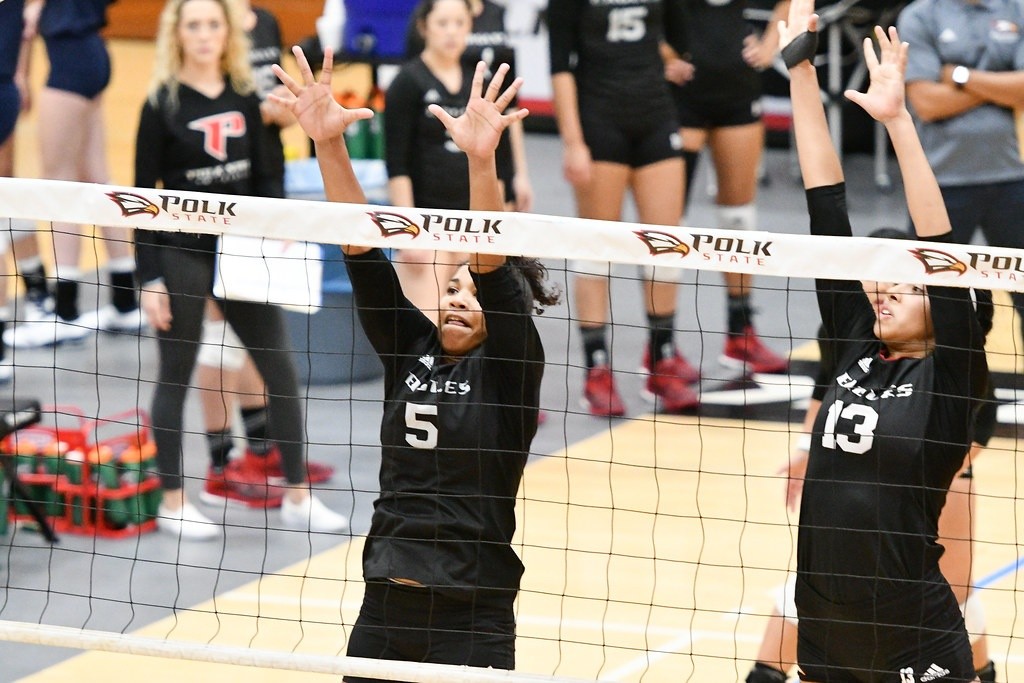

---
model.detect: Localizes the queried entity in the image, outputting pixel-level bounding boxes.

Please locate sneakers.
[720,325,789,375]
[204,456,287,506]
[641,343,702,383]
[643,370,701,414]
[244,442,331,483]
[580,365,626,417]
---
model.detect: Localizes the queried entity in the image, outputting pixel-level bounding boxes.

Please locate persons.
[0,0,1024,683]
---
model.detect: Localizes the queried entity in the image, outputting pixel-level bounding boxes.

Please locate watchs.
[949,67,970,89]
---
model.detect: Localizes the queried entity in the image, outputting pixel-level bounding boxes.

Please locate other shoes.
[281,495,348,532]
[156,505,220,539]
[0,297,147,349]
[0,300,147,377]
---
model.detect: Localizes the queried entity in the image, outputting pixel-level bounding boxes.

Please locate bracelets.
[798,435,811,451]
